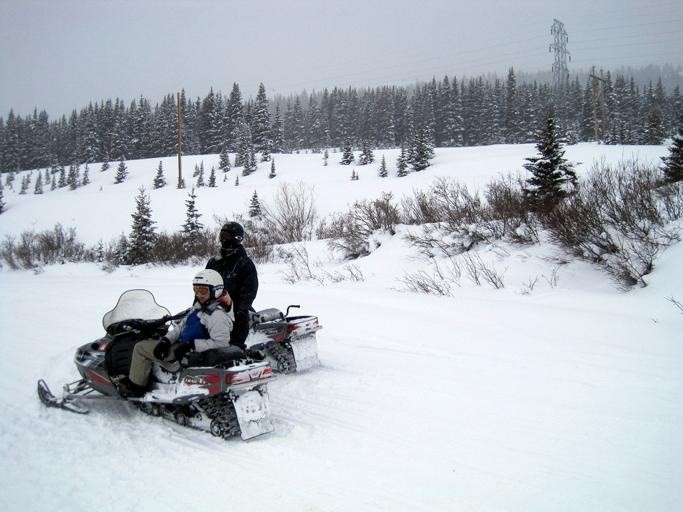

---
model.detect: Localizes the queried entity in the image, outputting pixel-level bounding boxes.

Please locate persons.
[206,222,259,356]
[107,268,232,397]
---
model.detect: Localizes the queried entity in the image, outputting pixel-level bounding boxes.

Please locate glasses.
[194,287,209,294]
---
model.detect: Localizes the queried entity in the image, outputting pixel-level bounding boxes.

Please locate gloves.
[174,342,192,360]
[153,337,171,360]
[235,311,247,322]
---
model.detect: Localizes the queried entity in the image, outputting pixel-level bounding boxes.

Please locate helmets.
[220,222,244,248]
[193,269,224,300]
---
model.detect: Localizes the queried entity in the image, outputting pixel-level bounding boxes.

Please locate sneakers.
[116,383,144,397]
